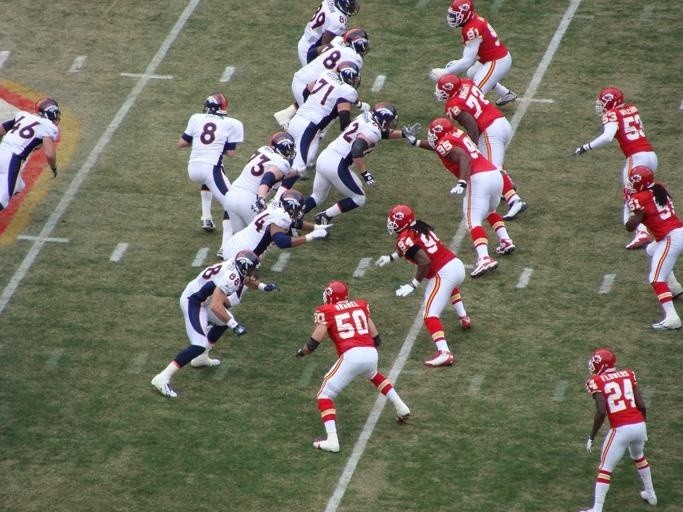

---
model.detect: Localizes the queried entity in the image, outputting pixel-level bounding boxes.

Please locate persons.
[578,350,658,512]
[623,166,683,330]
[575,87,659,226]
[0,98,61,212]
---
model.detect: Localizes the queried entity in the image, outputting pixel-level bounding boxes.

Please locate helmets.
[322,281,350,304]
[426,116,451,147]
[628,166,654,191]
[595,87,623,117]
[37,96,61,122]
[588,346,616,374]
[271,0,424,237]
[234,252,263,280]
[446,0,476,28]
[203,93,226,116]
[434,75,459,103]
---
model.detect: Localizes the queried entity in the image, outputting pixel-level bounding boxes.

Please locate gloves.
[49,166,60,180]
[375,253,394,267]
[297,345,311,358]
[396,281,416,298]
[586,435,594,456]
[450,179,467,195]
[264,282,281,293]
[575,143,593,156]
[232,323,248,335]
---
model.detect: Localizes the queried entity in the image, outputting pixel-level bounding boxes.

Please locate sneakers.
[492,90,516,106]
[394,407,411,424]
[625,231,681,332]
[502,197,525,219]
[641,491,657,505]
[424,350,455,368]
[470,257,498,277]
[313,439,342,453]
[496,239,515,254]
[192,357,222,369]
[202,216,216,232]
[459,316,473,329]
[150,374,178,397]
